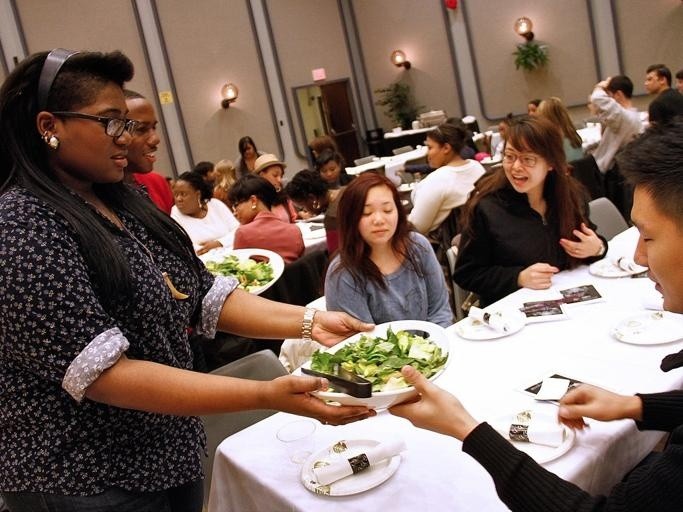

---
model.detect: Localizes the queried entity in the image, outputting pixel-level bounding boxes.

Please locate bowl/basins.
[290,320,454,410]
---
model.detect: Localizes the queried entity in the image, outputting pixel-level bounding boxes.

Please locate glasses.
[500,151,543,166]
[232,199,249,211]
[50,111,140,138]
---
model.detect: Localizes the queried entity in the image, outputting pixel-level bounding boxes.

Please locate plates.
[491,412,576,464]
[456,312,526,340]
[590,257,644,278]
[201,248,285,293]
[613,310,683,346]
[302,437,402,496]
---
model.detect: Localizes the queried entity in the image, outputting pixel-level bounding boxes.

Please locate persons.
[588,62,681,173]
[0,44,376,508]
[406,117,485,233]
[122,87,175,215]
[453,115,609,307]
[387,111,683,507]
[494,96,587,168]
[171,135,357,265]
[323,174,453,330]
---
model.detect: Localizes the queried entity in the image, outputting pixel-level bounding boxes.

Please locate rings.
[321,419,328,425]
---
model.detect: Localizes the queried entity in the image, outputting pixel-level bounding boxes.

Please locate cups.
[276,420,316,463]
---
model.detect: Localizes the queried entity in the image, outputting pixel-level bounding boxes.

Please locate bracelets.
[301,307,318,342]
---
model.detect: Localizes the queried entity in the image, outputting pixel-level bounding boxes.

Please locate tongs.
[303,359,375,399]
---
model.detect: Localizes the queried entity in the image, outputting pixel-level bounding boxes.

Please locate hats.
[253,154,287,174]
